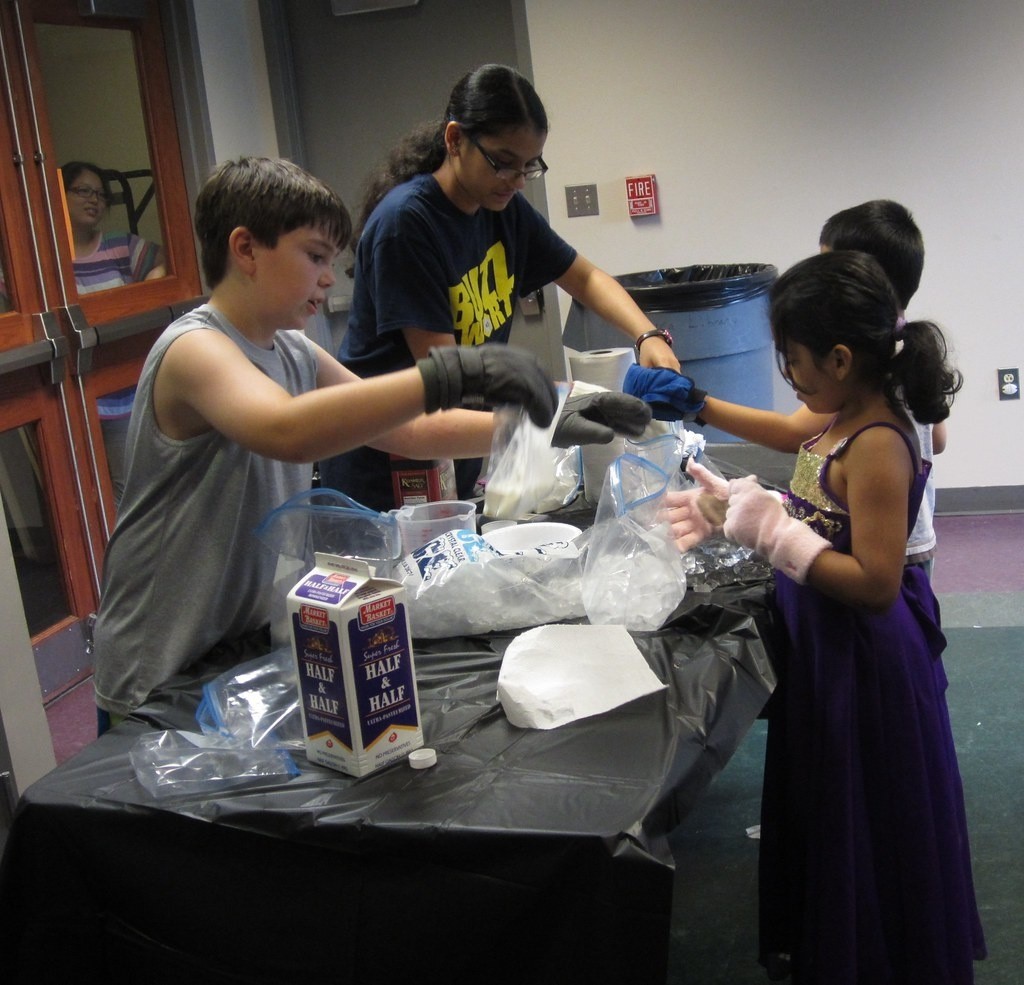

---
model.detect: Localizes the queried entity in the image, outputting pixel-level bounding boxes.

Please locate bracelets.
[634,328,674,364]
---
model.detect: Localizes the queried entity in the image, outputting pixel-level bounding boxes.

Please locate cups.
[481,520,517,535]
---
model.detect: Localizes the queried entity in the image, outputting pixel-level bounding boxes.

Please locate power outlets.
[998,368,1020,402]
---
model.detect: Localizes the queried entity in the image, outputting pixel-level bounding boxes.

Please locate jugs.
[395,500,477,555]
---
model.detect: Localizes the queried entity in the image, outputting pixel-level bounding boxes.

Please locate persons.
[94,155,652,739]
[622,199,947,585]
[61,161,167,510]
[665,249,990,985]
[318,65,682,516]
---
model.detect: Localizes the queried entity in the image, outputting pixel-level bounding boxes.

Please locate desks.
[0,563,778,983]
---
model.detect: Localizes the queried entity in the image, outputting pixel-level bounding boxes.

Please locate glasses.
[467,132,548,182]
[69,185,113,201]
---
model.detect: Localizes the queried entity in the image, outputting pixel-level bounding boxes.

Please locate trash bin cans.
[582,263,778,443]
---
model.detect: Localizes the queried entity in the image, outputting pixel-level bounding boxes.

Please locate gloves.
[622,362,708,428]
[551,380,653,449]
[722,477,833,586]
[416,341,559,428]
[657,454,784,554]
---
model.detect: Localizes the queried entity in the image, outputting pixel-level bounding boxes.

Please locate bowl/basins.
[481,522,582,550]
